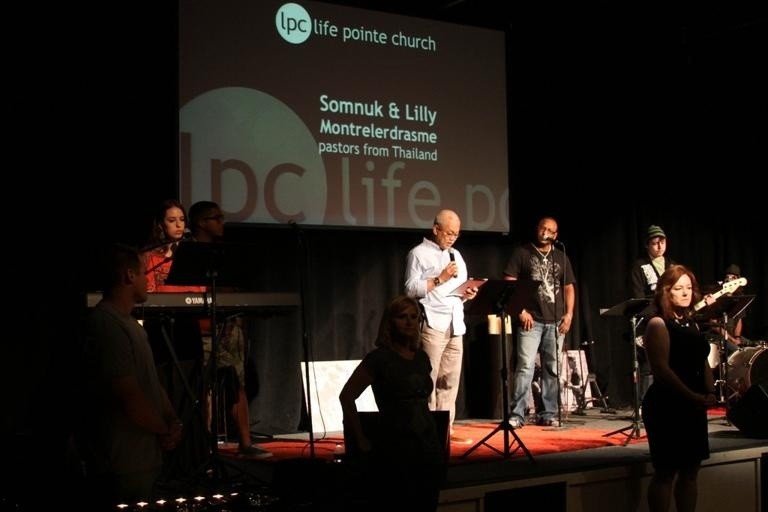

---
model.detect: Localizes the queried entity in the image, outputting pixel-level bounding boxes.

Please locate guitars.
[635,277,746,349]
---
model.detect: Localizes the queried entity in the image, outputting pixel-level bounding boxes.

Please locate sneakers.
[509,418,522,429]
[451,432,470,445]
[234,445,273,459]
[537,419,563,427]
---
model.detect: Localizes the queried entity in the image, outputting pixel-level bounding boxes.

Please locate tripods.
[577,334,616,417]
[603,317,647,447]
[461,312,539,471]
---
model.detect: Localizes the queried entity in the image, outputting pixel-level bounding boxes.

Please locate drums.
[721,345,768,437]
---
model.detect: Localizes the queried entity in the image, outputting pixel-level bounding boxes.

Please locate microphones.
[540,233,566,253]
[287,216,310,246]
[181,225,199,250]
[445,244,458,276]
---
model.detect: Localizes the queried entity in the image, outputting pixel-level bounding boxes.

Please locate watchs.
[433,277,440,285]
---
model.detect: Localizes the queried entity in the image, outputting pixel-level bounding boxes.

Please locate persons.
[403,209,479,436]
[500,215,576,430]
[630,224,678,403]
[697,263,744,407]
[133,200,200,417]
[86,244,183,511]
[642,264,717,512]
[164,200,274,458]
[339,296,442,511]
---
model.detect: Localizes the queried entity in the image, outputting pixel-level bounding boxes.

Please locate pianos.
[84,292,302,311]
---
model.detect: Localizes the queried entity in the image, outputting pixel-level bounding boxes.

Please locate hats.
[646,225,665,239]
[724,265,739,276]
